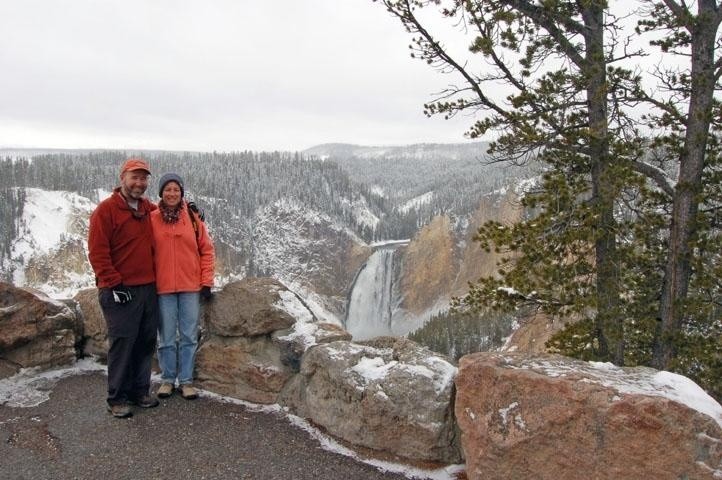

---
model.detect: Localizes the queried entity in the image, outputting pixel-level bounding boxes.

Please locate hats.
[120,159,152,176]
[159,173,184,198]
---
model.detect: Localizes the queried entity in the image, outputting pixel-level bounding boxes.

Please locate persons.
[88,159,215,418]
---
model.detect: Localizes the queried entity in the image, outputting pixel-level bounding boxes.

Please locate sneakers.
[177,383,197,399]
[105,395,159,416]
[157,382,175,397]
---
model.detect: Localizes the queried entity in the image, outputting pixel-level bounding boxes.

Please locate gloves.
[112,285,135,304]
[187,202,206,222]
[201,286,211,301]
